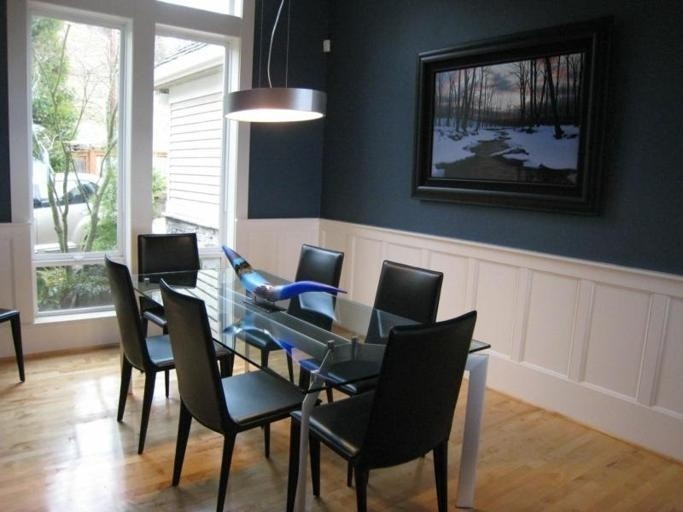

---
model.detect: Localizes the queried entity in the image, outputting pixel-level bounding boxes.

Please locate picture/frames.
[408,17,610,220]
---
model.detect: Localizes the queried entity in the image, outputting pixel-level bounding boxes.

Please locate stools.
[1,305,26,385]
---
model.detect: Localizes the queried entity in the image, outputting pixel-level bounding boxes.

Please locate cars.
[32,170,118,255]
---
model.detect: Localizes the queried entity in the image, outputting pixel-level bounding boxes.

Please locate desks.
[125,265,491,511]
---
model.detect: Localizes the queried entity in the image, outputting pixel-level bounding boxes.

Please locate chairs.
[134,231,207,397]
[221,240,346,405]
[157,274,324,510]
[290,312,479,511]
[104,254,236,454]
[299,258,444,487]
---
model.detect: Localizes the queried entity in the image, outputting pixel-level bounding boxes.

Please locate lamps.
[221,1,330,126]
